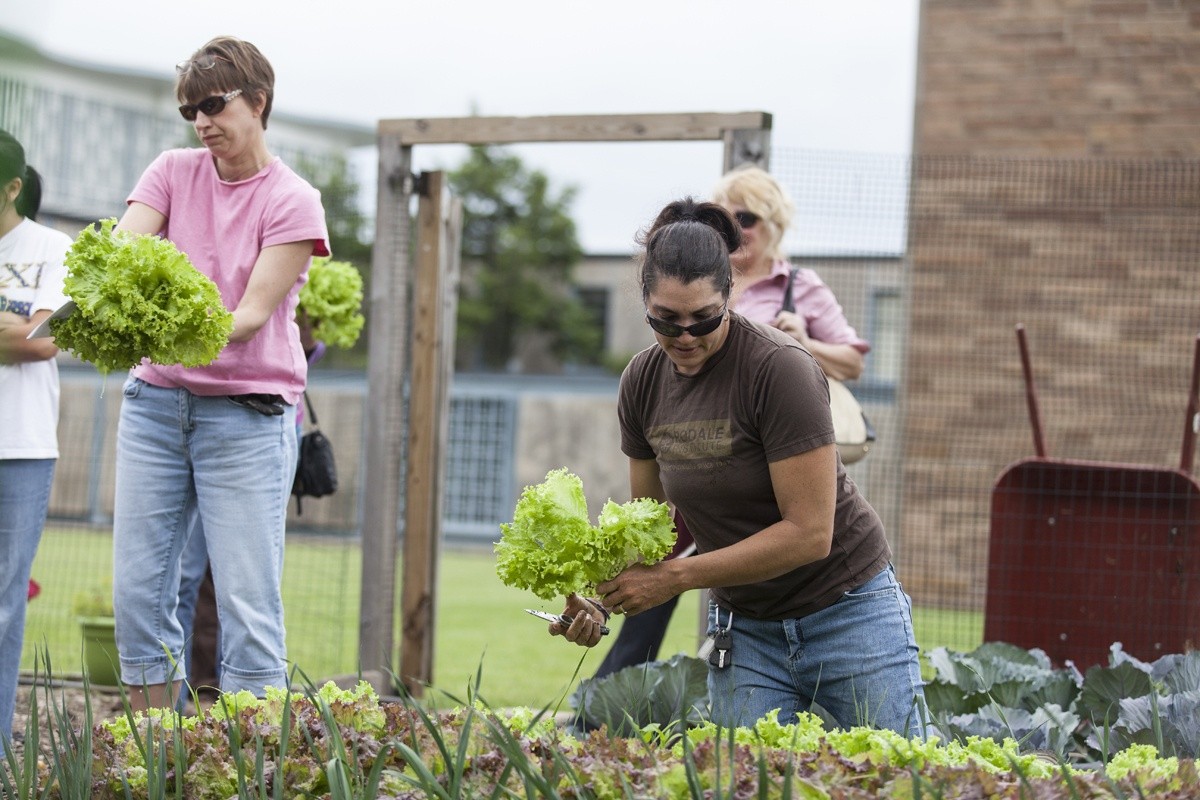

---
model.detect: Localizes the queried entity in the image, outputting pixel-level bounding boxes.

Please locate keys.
[715,633,732,668]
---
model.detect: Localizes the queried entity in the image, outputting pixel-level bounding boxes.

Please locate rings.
[620,604,627,614]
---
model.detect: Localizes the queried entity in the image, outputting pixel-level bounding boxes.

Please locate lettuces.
[492,467,676,602]
[652,708,1200,800]
[49,218,234,368]
[99,681,619,800]
[297,251,366,350]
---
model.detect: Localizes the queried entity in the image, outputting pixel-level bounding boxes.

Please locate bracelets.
[586,598,611,619]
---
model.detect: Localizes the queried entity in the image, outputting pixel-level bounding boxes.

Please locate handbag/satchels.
[824,376,879,465]
[290,426,341,517]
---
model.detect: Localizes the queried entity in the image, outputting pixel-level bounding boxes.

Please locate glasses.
[178,89,242,122]
[732,209,765,228]
[644,283,735,339]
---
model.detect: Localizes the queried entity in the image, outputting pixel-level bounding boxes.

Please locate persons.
[111,37,331,718]
[548,201,934,746]
[0,130,75,757]
[595,167,872,677]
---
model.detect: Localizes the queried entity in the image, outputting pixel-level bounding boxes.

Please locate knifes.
[524,609,610,635]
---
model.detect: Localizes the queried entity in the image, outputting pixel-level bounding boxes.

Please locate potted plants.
[75,592,127,687]
